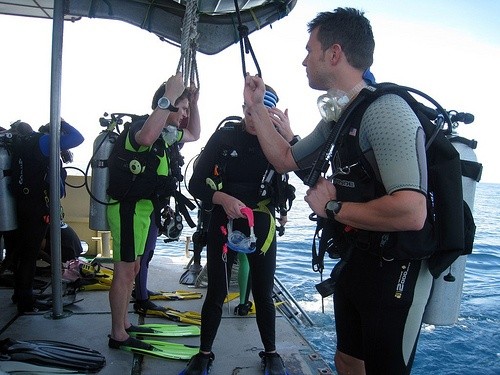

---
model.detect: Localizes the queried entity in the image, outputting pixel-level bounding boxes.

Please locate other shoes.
[178,350,216,375]
[258,350,290,375]
[11,294,54,315]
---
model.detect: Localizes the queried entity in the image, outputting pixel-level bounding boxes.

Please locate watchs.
[288,135,301,146]
[157,97,180,113]
[324,199,342,222]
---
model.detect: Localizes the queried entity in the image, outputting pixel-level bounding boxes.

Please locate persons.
[243,6,429,375]
[107,73,168,355]
[0,116,83,315]
[179,209,209,289]
[178,84,321,375]
